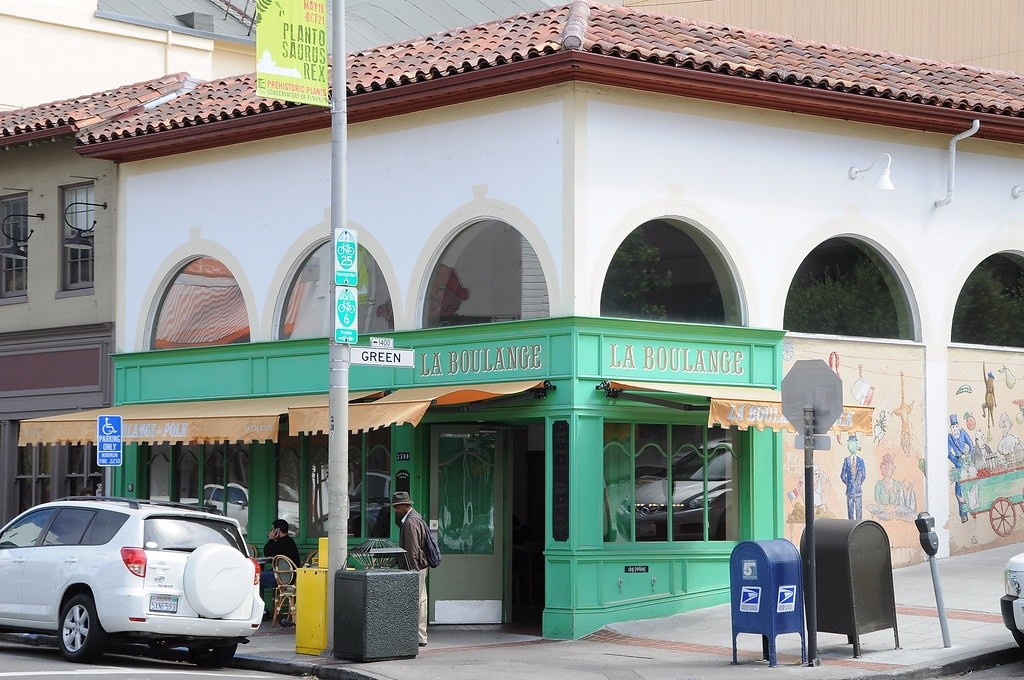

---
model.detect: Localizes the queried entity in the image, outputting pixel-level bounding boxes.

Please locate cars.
[616,437,739,542]
[151,482,299,534]
[1000,553,1024,648]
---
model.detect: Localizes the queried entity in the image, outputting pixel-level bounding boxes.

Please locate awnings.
[609,380,874,436]
[288,380,547,437]
[17,390,386,447]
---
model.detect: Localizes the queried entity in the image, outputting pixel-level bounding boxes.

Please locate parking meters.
[915,512,951,648]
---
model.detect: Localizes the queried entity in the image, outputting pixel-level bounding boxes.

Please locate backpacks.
[404,514,442,568]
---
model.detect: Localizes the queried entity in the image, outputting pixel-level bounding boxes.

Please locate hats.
[387,491,415,506]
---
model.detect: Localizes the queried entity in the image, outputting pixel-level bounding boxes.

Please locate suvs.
[0,495,265,668]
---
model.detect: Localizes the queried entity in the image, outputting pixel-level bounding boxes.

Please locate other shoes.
[418,643,427,647]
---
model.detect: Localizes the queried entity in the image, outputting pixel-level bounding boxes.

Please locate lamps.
[64,202,107,249]
[849,153,895,190]
[0,213,43,260]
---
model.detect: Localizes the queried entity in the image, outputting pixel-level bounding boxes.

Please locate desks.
[249,555,305,621]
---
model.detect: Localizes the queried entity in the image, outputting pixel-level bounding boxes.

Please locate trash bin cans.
[799,517,903,660]
[294,538,354,656]
[729,537,807,669]
[334,539,419,662]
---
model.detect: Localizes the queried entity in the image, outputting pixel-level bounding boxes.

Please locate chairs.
[270,549,319,629]
[245,543,258,558]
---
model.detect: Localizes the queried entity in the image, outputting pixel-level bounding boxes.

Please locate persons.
[386,492,429,647]
[259,519,300,620]
